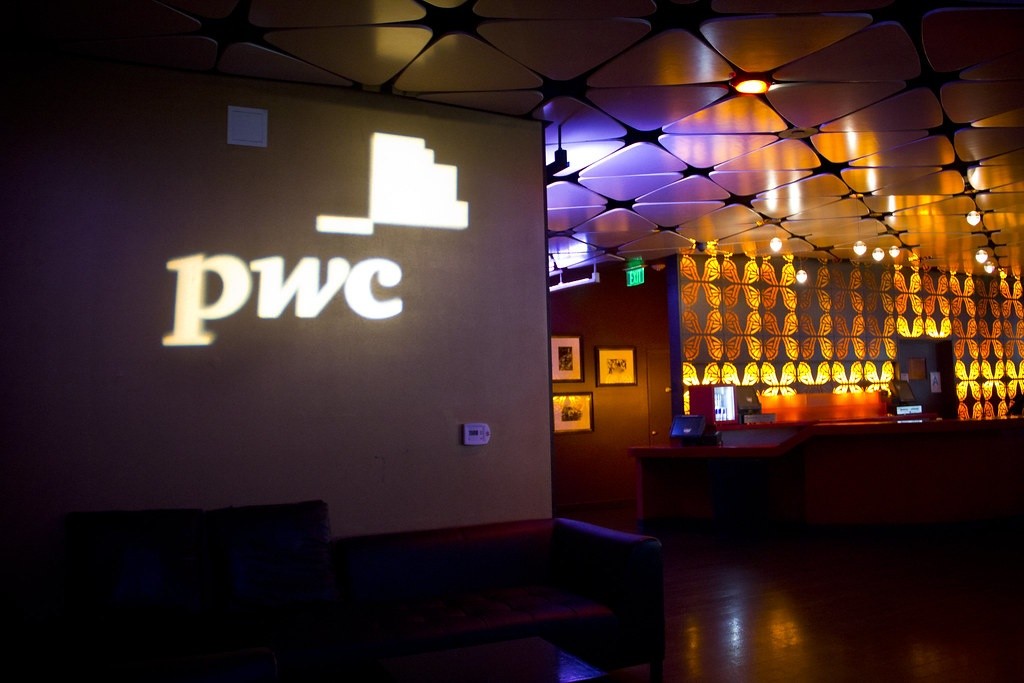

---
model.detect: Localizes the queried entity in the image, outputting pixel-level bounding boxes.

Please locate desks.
[270,635,607,683]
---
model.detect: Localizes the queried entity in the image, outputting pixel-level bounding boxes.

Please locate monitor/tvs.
[735,385,761,409]
[889,379,917,402]
[669,415,705,437]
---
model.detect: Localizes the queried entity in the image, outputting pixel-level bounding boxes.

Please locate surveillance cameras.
[695,243,707,251]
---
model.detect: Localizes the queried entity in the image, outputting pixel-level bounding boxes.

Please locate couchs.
[0,499,664,683]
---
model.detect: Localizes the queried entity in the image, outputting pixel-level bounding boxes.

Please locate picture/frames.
[550,335,585,382]
[927,369,943,395]
[553,392,594,434]
[907,357,928,381]
[595,345,637,386]
[900,371,910,386]
